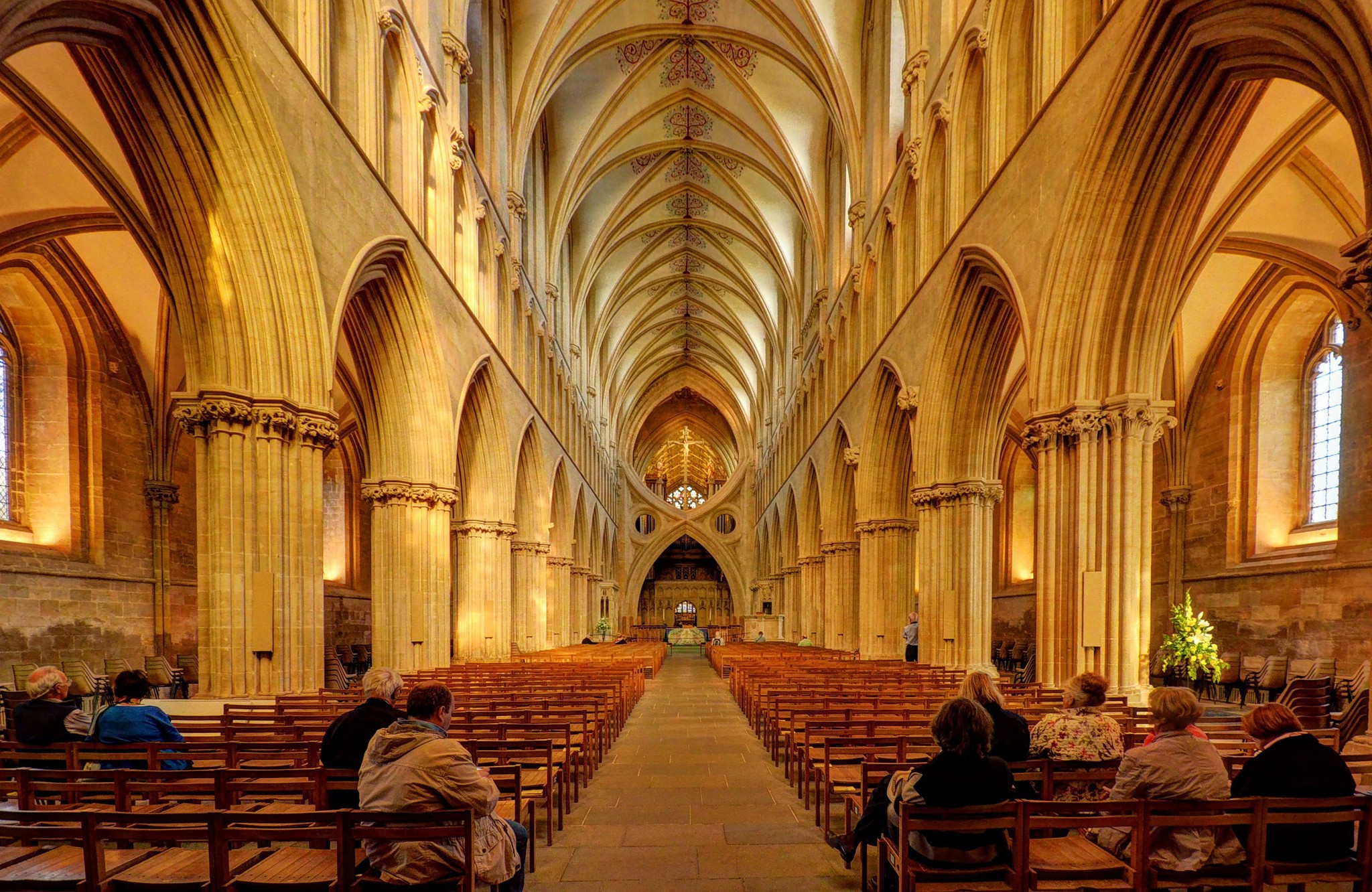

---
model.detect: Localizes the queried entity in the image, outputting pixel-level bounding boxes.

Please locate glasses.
[1061,692,1071,698]
[398,688,402,697]
[58,679,74,688]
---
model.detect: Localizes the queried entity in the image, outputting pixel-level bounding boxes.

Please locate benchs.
[0,661,647,892]
[729,659,1371,892]
[700,635,862,681]
[511,641,672,682]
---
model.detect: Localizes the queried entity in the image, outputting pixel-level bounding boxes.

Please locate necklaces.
[115,699,142,707]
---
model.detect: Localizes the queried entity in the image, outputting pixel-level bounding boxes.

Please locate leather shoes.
[824,831,856,862]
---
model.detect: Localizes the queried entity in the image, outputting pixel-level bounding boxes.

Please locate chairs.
[990,634,1038,696]
[321,643,373,694]
[1,651,201,737]
[1145,641,1372,744]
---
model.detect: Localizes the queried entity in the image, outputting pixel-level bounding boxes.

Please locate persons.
[578,621,824,653]
[902,612,919,663]
[656,438,718,482]
[823,696,1017,892]
[84,671,202,805]
[1076,686,1250,880]
[1027,668,1125,839]
[358,681,529,892]
[12,665,95,795]
[1224,702,1357,874]
[1141,687,1211,751]
[320,663,412,810]
[957,670,1041,818]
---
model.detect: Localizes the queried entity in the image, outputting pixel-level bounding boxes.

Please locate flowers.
[1156,591,1232,684]
